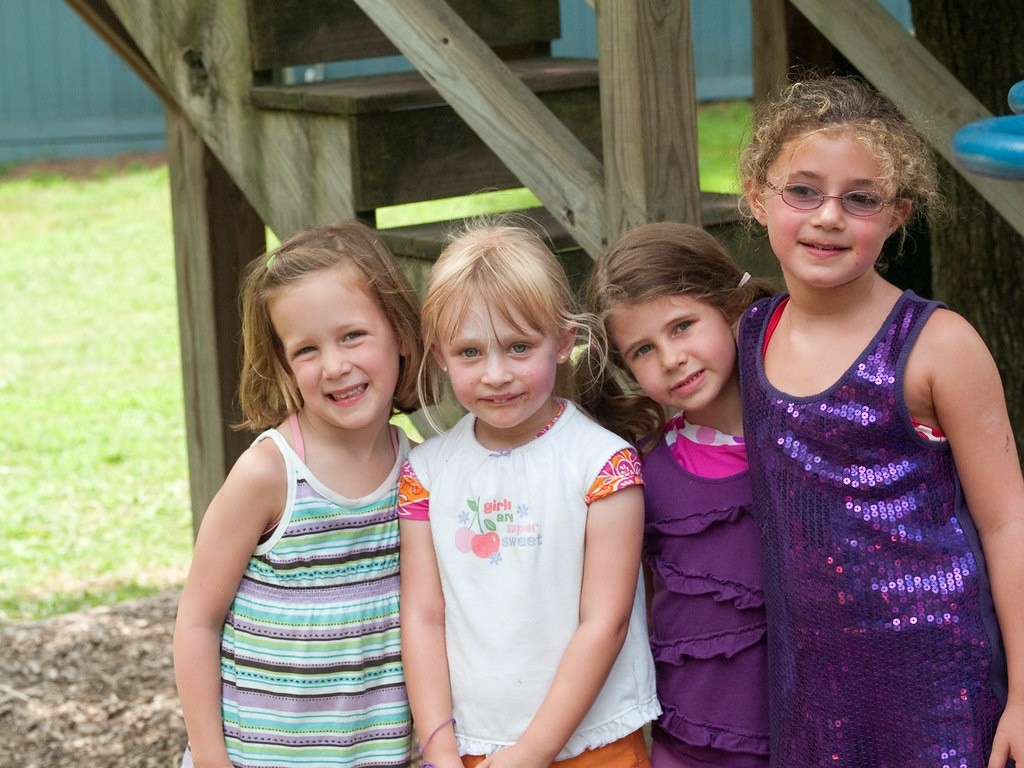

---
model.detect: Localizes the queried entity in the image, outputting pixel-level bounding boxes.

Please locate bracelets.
[419,719,456,755]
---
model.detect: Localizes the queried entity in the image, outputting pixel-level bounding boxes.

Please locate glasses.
[761,176,899,217]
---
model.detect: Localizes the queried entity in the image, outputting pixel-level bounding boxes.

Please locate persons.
[573,222,772,767]
[730,63,1024,766]
[171,219,443,765]
[397,212,661,768]
